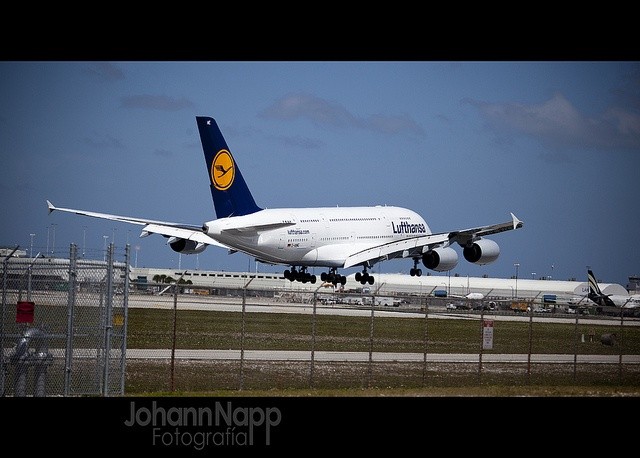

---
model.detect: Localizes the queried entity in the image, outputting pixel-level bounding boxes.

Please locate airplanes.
[586,265,640,310]
[446,288,512,312]
[45,116,524,285]
